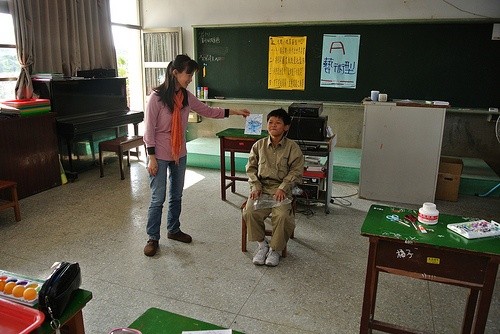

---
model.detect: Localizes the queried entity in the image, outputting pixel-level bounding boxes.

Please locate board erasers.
[215,96,226,100]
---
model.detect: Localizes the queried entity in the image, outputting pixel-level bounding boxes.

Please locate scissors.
[404,214,419,231]
[386,214,411,228]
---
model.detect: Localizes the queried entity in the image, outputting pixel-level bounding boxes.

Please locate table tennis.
[0,280,7,291]
[12,285,26,297]
[23,287,37,300]
[4,281,16,295]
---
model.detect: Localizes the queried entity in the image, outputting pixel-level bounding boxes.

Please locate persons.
[243,109,306,266]
[143,55,250,257]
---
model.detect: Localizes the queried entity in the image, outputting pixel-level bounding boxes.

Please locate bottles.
[197,87,208,99]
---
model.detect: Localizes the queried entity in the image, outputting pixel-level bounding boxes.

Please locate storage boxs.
[435,157,463,200]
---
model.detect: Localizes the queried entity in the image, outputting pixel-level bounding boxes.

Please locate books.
[31,72,65,80]
[0,99,52,116]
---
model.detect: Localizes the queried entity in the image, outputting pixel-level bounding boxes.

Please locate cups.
[371,91,379,101]
[378,94,387,102]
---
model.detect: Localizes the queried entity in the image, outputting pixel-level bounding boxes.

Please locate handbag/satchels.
[38,261,81,319]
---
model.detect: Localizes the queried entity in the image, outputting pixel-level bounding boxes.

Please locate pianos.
[31,68,144,181]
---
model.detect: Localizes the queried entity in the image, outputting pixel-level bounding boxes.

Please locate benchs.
[98,136,148,180]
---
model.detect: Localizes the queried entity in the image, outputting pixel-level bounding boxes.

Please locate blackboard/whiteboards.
[190,17,500,116]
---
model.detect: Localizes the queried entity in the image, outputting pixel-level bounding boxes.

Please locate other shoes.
[167,230,192,243]
[144,239,159,256]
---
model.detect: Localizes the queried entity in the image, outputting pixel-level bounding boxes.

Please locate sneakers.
[253,240,269,265]
[265,247,282,267]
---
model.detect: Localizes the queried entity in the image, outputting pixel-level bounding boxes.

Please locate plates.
[0,273,42,307]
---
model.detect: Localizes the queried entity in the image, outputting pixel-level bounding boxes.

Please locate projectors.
[288,103,324,118]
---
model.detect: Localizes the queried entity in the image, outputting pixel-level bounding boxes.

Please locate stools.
[302,165,325,199]
[0,180,20,221]
[240,197,298,258]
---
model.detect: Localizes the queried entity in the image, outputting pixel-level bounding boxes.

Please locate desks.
[0,269,92,334]
[121,307,246,334]
[216,128,336,213]
[360,205,500,334]
